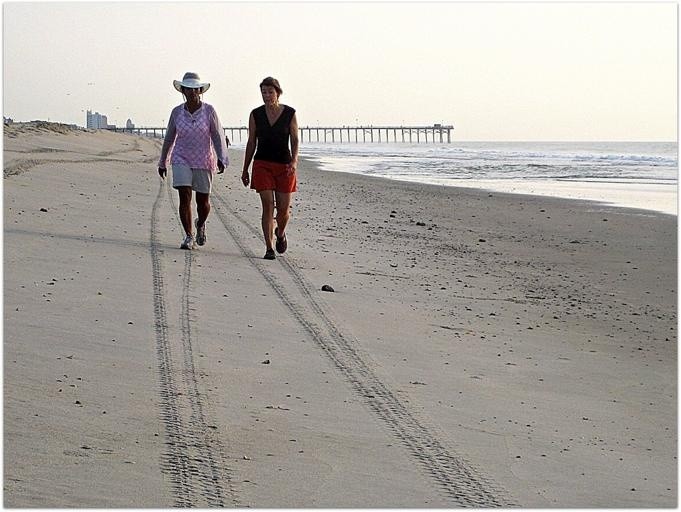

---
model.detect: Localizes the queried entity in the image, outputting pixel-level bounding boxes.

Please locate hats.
[174,72,210,94]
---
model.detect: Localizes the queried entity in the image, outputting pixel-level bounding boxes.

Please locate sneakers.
[275,227,287,253]
[264,251,276,259]
[181,237,197,250]
[195,218,206,246]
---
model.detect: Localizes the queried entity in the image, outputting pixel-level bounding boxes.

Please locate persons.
[225,136,230,148]
[240,77,298,260]
[158,71,229,249]
